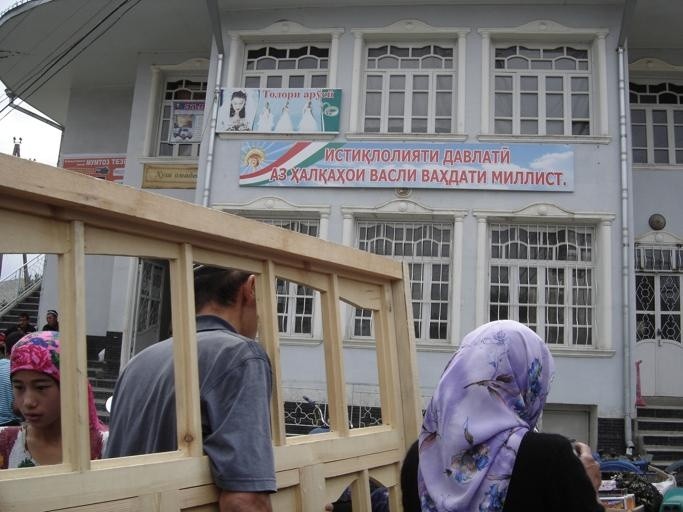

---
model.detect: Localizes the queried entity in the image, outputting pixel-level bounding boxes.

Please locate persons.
[307,428,390,512]
[399,317,606,511]
[0,313,37,427]
[256,102,273,132]
[274,101,293,133]
[298,100,319,132]
[0,329,112,471]
[103,263,335,512]
[218,91,251,133]
[41,309,58,332]
